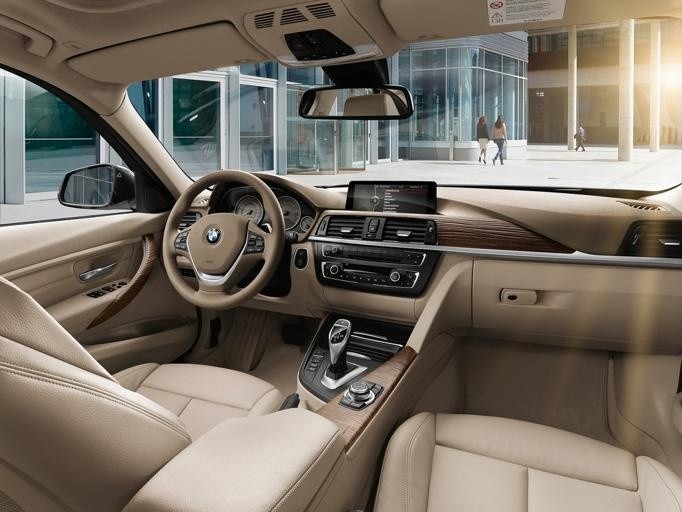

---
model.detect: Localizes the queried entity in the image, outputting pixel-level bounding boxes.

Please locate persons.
[492,116,508,166]
[575,124,586,152]
[475,116,491,164]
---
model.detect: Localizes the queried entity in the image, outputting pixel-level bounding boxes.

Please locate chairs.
[0,275,282,512]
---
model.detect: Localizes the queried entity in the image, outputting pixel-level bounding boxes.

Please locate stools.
[374,409,681,512]
